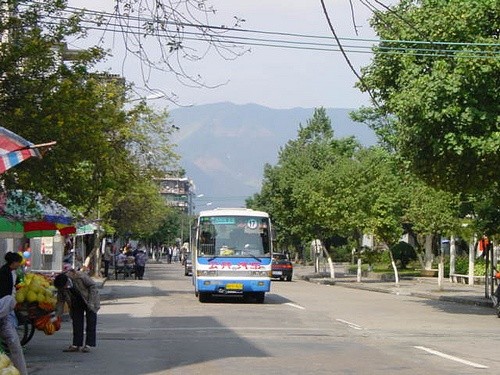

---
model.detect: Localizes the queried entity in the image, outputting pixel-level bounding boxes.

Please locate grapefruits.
[16,280,57,307]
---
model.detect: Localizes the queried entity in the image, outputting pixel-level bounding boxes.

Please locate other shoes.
[63,345,79,352]
[81,347,90,352]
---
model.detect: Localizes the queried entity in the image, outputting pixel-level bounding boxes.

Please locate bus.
[191,208,273,304]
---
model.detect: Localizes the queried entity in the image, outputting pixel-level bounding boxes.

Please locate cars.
[183,252,192,276]
[271,253,293,281]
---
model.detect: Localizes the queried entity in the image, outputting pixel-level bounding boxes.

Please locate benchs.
[473,276,493,284]
[449,274,469,284]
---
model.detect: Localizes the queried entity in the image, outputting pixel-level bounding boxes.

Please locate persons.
[0,250,23,299]
[105,242,116,277]
[116,241,189,280]
[49,268,100,353]
